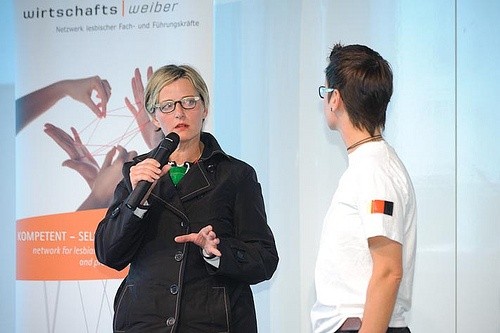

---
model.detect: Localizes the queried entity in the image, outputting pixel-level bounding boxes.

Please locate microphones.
[126,132,180,212]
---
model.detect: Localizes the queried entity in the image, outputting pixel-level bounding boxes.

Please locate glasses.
[319,85,343,100]
[152,95,201,113]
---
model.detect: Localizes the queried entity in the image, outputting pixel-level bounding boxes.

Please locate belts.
[338,317,361,330]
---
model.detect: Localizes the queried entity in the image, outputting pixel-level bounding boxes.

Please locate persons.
[16,65,163,213]
[309,43,418,333]
[94,65,280,333]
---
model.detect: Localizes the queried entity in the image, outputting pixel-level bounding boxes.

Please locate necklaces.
[346,134,383,151]
